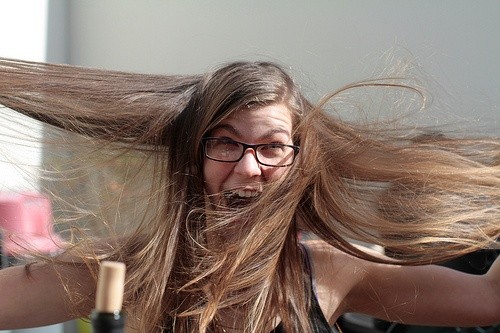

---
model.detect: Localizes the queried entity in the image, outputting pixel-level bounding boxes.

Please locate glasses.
[200,136,300,167]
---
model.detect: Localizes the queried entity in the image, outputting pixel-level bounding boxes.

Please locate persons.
[0,58,500,333]
[371,132,500,333]
